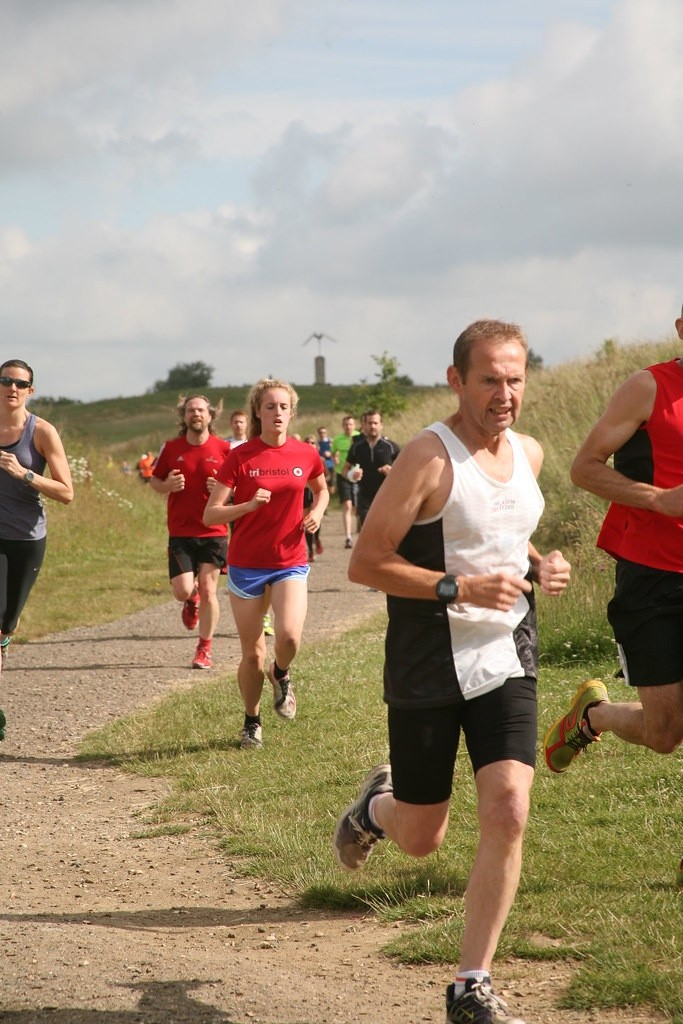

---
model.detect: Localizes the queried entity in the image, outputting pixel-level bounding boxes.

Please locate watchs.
[21,469,35,486]
[436,573,460,603]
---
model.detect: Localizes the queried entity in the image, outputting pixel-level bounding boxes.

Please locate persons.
[329,317,573,1024]
[223,410,252,532]
[137,450,158,486]
[150,393,239,671]
[329,415,364,549]
[341,409,401,594]
[261,582,276,637]
[202,376,332,750]
[540,305,682,888]
[0,357,76,740]
[299,433,332,563]
[290,424,337,496]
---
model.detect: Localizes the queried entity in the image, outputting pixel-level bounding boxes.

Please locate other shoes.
[307,551,314,562]
[314,539,323,555]
[0,638,11,669]
[344,538,354,548]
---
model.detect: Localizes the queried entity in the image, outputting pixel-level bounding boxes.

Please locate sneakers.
[181,581,200,631]
[445,976,524,1024]
[265,662,297,719]
[544,680,611,774]
[240,723,262,750]
[332,765,394,872]
[192,645,211,669]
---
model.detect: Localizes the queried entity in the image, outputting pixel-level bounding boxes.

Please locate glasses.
[305,441,316,445]
[0,376,31,389]
